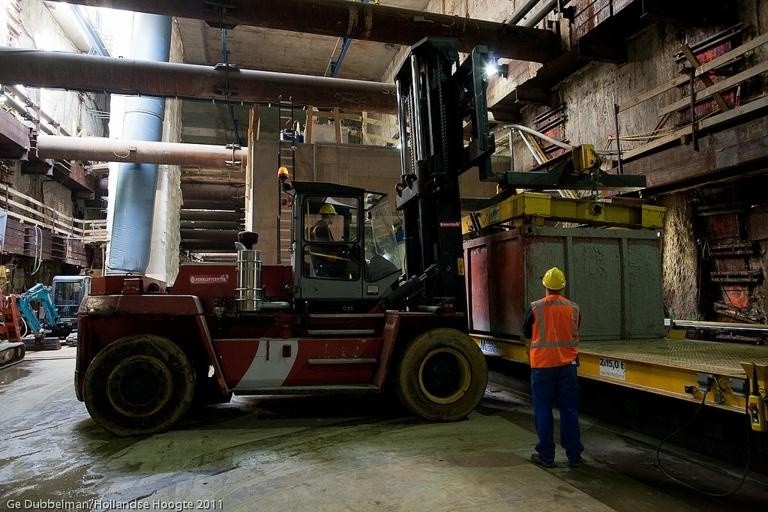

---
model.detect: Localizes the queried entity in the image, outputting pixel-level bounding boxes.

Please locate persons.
[308,204,362,281]
[521,266,588,468]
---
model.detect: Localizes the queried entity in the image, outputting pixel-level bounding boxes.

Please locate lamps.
[486,63,508,78]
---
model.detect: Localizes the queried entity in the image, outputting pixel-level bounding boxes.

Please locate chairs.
[306,227,346,278]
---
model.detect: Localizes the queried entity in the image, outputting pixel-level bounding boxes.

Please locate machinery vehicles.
[0,275,92,370]
[75,37,648,437]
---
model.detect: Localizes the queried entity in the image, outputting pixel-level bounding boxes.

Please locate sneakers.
[568,459,585,469]
[531,453,553,468]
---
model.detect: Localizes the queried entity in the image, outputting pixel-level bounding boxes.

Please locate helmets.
[318,204,338,214]
[542,267,567,290]
